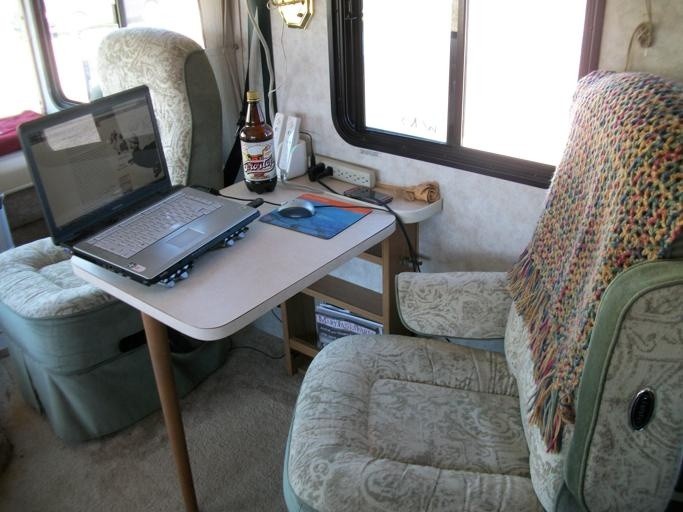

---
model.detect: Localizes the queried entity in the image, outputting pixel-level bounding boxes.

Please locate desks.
[69,179,399,512]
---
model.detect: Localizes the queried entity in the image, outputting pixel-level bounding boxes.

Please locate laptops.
[16,84,261,287]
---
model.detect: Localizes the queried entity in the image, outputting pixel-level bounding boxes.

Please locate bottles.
[238,90,278,192]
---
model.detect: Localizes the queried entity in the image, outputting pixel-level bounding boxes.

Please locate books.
[315,303,384,350]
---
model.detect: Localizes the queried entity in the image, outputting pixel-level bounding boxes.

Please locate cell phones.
[344,185,393,206]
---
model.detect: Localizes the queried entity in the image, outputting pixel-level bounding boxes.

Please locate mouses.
[278,199,315,218]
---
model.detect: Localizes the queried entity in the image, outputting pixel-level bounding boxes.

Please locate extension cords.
[308,154,376,189]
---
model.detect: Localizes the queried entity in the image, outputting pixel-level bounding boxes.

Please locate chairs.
[1,26,223,444]
[281,74,682,512]
